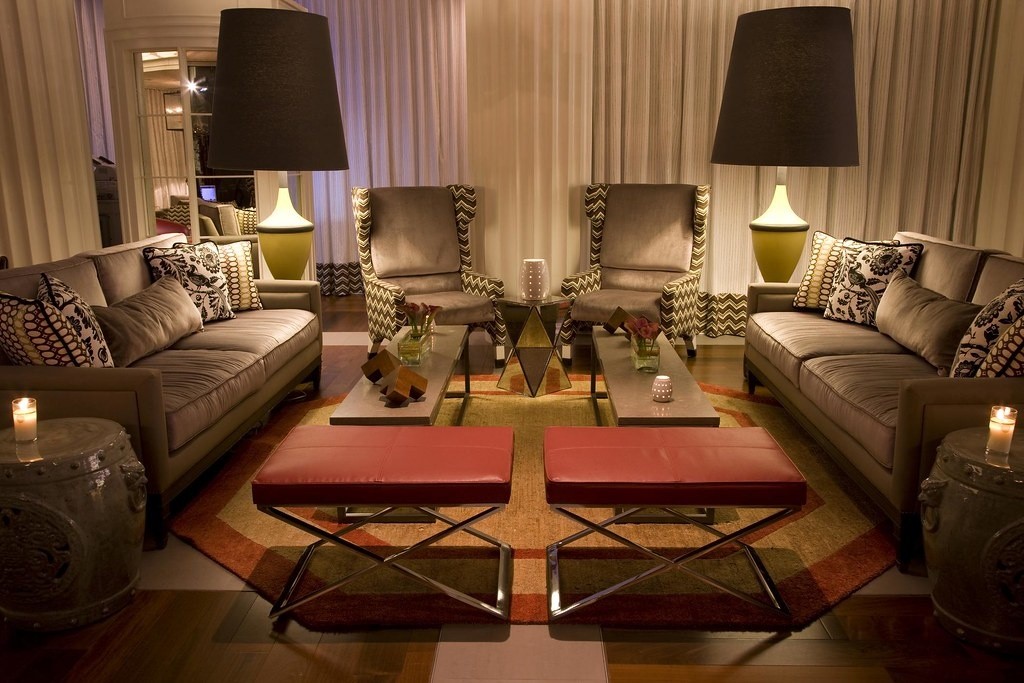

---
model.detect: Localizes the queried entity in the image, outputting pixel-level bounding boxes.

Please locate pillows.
[163,205,191,231]
[237,209,257,235]
[793,231,1024,378]
[0,241,263,367]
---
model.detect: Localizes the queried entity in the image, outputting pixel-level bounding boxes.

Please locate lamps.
[520,259,550,300]
[711,6,860,281]
[207,7,349,281]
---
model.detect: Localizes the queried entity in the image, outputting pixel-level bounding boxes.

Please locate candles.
[987,409,1015,454]
[13,399,37,440]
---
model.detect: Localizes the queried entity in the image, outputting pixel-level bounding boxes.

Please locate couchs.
[170,195,241,236]
[743,231,1024,574]
[0,233,324,549]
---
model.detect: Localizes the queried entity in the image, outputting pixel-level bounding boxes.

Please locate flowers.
[626,317,659,357]
[400,302,442,340]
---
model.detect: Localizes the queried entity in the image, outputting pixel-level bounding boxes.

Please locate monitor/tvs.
[201,185,217,202]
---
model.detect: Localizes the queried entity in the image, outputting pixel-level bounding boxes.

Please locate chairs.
[350,184,710,368]
[156,218,190,237]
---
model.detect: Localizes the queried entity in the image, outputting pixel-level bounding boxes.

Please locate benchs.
[251,424,806,618]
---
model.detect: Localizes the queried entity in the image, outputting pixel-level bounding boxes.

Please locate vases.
[398,329,433,367]
[631,335,660,374]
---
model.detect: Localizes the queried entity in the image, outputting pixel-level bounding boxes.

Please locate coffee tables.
[330,326,720,521]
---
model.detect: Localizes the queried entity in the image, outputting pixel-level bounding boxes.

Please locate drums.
[0,415,151,636]
[918,426,1024,658]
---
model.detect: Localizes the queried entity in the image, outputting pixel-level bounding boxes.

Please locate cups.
[985,405,1017,456]
[12,397,37,443]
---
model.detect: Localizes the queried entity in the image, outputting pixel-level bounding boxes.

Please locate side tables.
[498,295,570,398]
[0,418,149,629]
[918,428,1024,656]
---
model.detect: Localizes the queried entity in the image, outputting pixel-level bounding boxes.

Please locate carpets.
[170,374,899,631]
